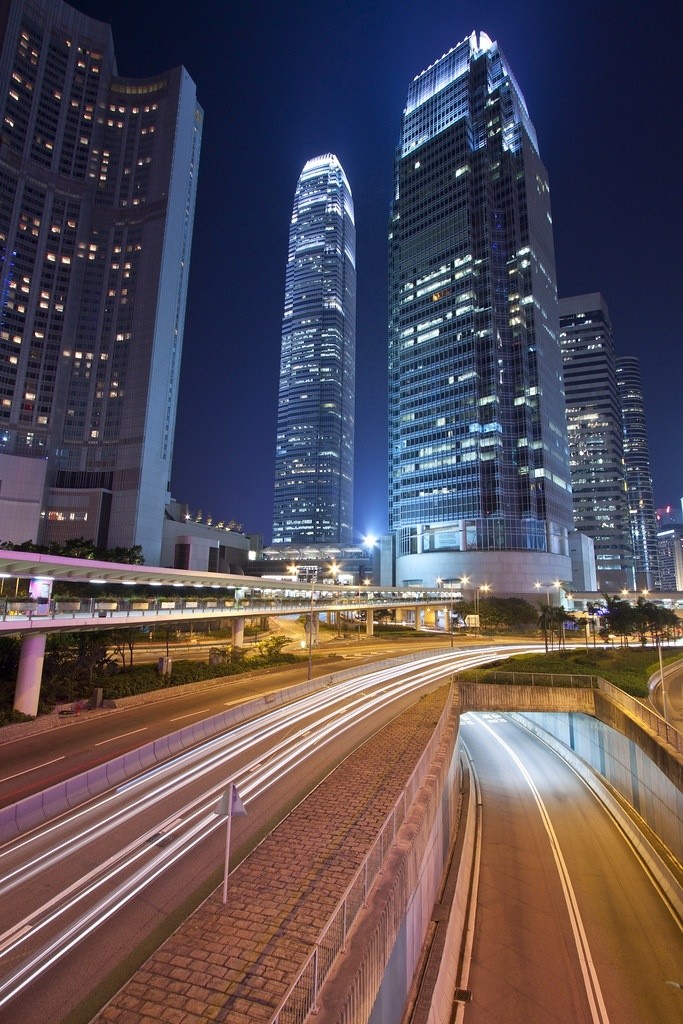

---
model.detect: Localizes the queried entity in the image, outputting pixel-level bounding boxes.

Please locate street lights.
[476,585,491,634]
[533,579,561,642]
[358,566,371,642]
[285,564,339,681]
[621,588,650,606]
[434,576,470,647]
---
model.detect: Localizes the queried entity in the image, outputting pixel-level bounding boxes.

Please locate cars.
[452,620,466,631]
